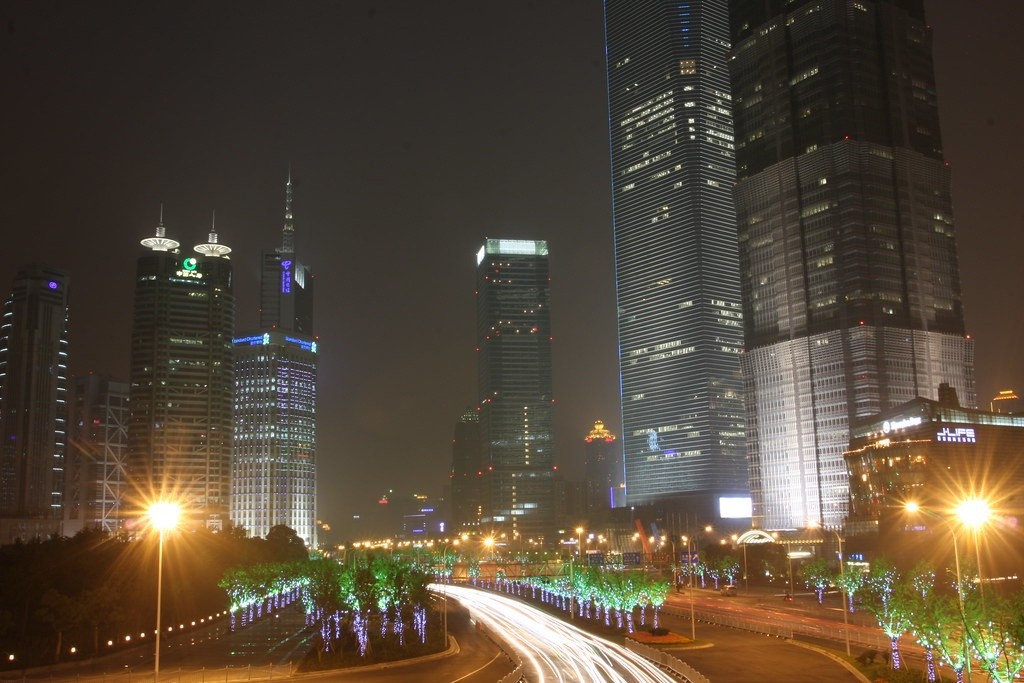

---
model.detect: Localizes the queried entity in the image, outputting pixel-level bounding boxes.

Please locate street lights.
[682,534,698,589]
[902,497,966,626]
[443,533,469,650]
[730,532,749,596]
[685,524,712,641]
[521,539,533,561]
[143,511,182,683]
[951,497,998,626]
[660,536,678,592]
[804,523,851,657]
[631,532,644,566]
[588,534,606,568]
[514,532,522,544]
[417,541,433,569]
[484,535,495,564]
[575,527,584,573]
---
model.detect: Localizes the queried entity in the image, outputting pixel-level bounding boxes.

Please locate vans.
[721,585,736,596]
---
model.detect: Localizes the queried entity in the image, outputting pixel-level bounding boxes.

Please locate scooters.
[784,592,792,602]
